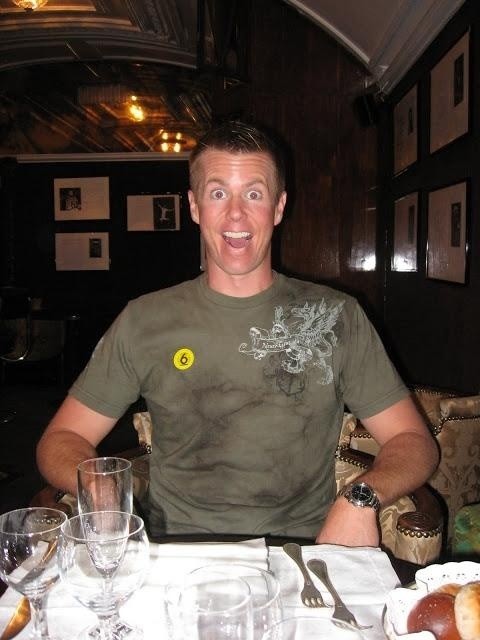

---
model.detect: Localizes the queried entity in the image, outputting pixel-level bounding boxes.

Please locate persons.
[33,118,441,547]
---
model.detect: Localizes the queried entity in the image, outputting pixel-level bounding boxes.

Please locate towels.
[32,537,266,640]
[267,544,403,628]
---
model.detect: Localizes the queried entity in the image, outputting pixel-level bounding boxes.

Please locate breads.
[406,592,463,640]
[437,584,462,596]
[453,580,480,640]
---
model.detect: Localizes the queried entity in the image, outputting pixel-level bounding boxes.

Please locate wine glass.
[0,455,154,640]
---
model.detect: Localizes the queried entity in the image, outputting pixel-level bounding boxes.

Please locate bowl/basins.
[382,578,417,640]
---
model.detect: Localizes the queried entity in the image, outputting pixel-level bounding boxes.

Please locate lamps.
[12,0,47,14]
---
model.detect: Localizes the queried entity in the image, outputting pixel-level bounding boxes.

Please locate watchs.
[333,481,380,513]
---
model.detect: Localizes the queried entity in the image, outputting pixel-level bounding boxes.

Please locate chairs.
[350,384,480,562]
[29,412,448,564]
[0,287,82,391]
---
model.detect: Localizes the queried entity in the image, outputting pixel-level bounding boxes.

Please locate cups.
[262,614,366,640]
[162,560,280,640]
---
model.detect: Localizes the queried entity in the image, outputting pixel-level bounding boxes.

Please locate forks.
[307,557,373,632]
[282,541,332,609]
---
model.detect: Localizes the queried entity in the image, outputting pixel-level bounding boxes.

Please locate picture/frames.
[429,27,472,161]
[390,189,422,274]
[391,80,424,181]
[425,177,470,288]
